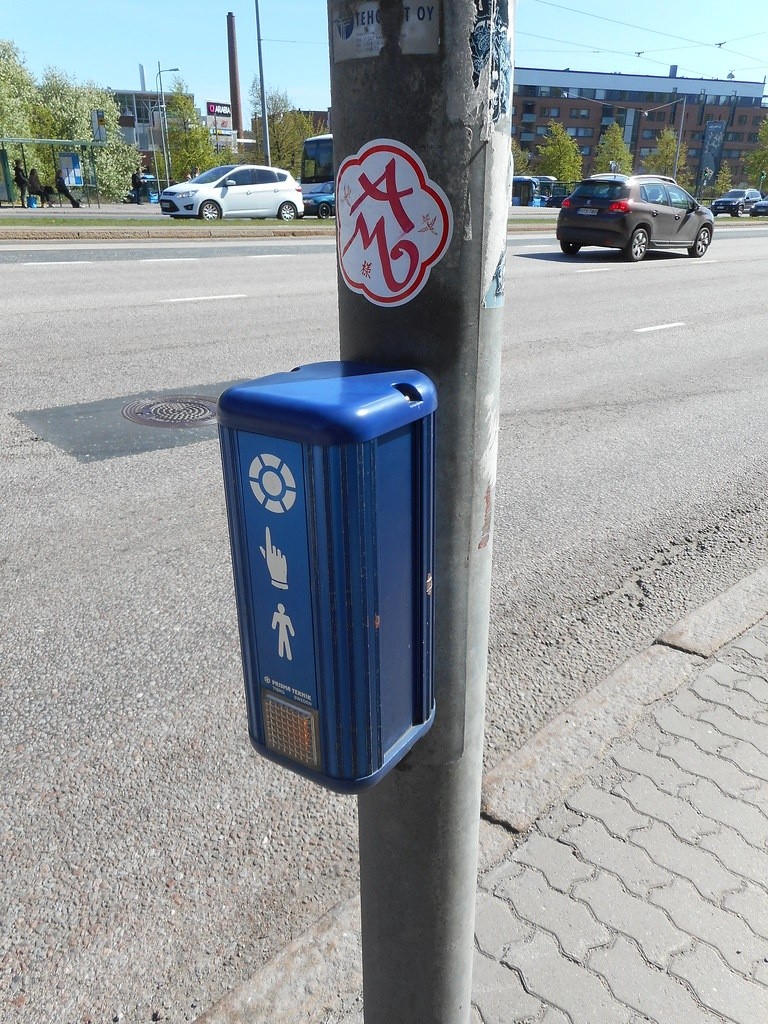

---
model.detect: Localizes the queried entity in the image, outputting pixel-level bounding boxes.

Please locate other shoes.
[49,205,55,208]
[41,205,47,208]
[78,204,85,208]
[138,202,144,205]
[22,203,27,208]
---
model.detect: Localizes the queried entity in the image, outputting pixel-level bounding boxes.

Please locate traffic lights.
[761,171,766,181]
[702,169,710,180]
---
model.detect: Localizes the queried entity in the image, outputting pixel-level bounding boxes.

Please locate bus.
[301,133,335,196]
[509,174,557,208]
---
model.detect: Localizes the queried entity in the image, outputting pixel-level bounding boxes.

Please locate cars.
[157,164,305,222]
[302,181,336,220]
[544,195,570,208]
[749,194,768,217]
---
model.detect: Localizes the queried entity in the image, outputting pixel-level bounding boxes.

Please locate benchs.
[28,192,66,207]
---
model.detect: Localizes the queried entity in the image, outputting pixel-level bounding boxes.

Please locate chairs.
[648,189,662,203]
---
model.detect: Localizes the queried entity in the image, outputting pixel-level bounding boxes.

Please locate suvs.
[710,188,762,218]
[556,171,714,262]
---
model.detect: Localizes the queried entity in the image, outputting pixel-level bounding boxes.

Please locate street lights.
[154,68,180,188]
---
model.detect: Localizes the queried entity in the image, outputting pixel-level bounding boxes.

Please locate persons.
[14,159,29,207]
[28,169,53,208]
[55,170,81,208]
[136,168,144,205]
